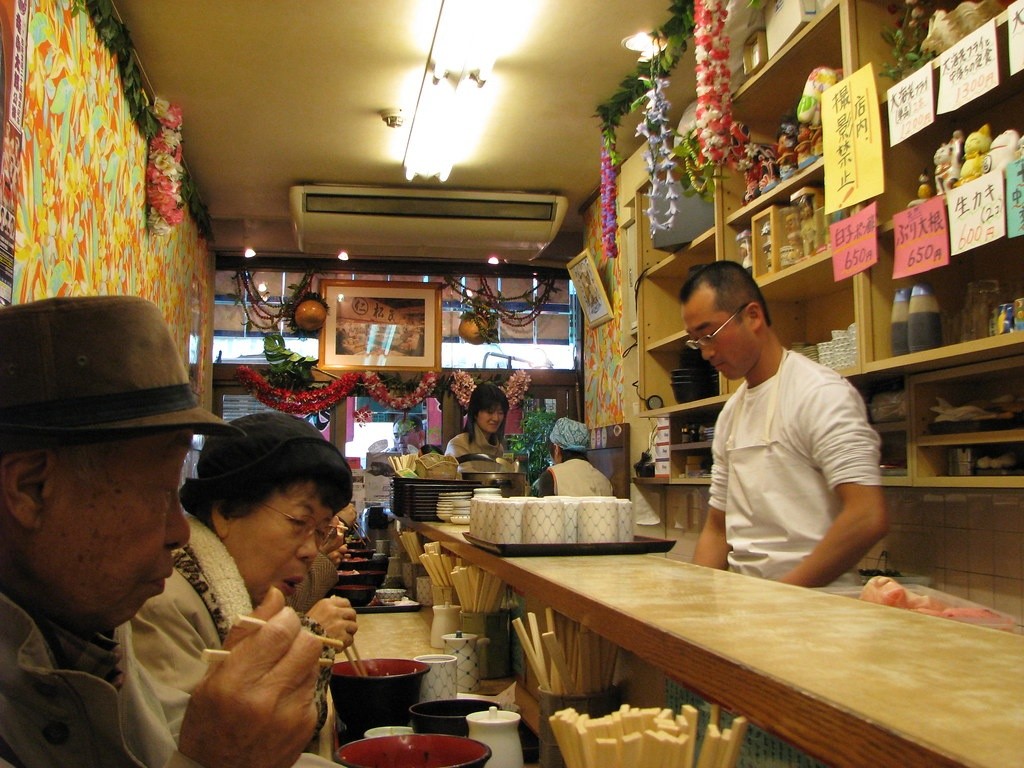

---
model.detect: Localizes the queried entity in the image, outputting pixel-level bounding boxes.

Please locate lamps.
[405,67,486,181]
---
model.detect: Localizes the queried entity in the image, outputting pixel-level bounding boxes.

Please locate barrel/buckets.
[461,472,527,498]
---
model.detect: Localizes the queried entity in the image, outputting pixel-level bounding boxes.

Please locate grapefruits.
[295,301,327,330]
[459,315,488,344]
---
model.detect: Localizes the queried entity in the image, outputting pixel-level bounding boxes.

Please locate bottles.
[430,604,462,648]
[889,282,944,355]
[440,632,482,692]
[465,709,524,768]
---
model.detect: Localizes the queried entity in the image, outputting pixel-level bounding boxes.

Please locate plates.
[436,491,474,524]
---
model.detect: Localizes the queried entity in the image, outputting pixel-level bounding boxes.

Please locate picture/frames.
[318,279,443,373]
[566,248,614,328]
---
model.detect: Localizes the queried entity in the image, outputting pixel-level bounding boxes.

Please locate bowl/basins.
[318,536,491,768]
[670,364,720,404]
[789,323,857,371]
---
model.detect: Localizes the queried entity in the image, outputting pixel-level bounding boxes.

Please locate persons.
[540,416,615,497]
[444,383,509,460]
[678,259,891,591]
[1,296,356,768]
[392,412,426,453]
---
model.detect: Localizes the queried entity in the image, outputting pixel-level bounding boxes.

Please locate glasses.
[685,303,752,349]
[250,499,337,549]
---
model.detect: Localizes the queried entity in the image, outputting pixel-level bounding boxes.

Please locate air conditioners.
[286,180,567,265]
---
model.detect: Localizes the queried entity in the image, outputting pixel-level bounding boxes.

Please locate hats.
[550,417,590,449]
[0,296,247,438]
[180,412,353,518]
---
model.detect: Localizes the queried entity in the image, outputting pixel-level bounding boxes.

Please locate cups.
[412,654,459,703]
[469,494,634,545]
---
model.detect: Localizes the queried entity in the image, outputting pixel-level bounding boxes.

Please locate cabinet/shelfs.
[630,0,1024,488]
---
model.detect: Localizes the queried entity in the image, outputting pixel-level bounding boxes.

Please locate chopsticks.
[200,613,345,667]
[328,594,368,677]
[399,528,749,767]
[387,453,418,476]
